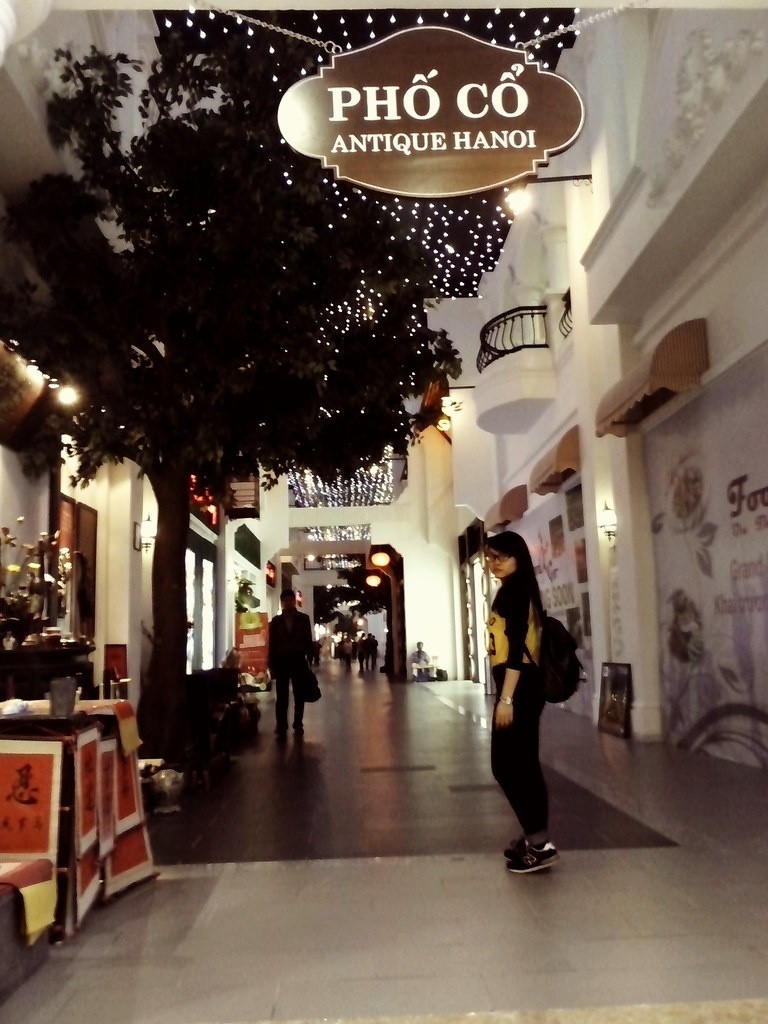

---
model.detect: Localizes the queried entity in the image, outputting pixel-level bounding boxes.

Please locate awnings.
[529,424,583,495]
[482,485,528,531]
[594,317,709,439]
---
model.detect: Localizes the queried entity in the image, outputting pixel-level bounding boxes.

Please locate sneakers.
[503,837,563,874]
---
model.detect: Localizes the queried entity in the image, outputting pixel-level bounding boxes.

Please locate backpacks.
[503,579,585,704]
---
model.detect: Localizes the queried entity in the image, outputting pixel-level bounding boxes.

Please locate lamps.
[133,515,157,554]
[597,500,618,551]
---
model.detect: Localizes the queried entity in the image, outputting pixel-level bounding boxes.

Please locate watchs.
[498,695,514,706]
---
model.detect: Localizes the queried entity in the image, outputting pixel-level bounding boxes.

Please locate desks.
[0,699,160,947]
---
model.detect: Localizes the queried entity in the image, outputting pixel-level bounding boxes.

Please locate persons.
[483,531,559,872]
[269,590,312,737]
[336,633,380,671]
[411,643,436,682]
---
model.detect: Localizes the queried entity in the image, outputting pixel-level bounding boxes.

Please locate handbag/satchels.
[304,667,322,702]
[436,668,448,681]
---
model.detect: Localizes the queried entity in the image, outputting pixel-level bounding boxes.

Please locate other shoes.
[293,727,305,738]
[274,727,287,741]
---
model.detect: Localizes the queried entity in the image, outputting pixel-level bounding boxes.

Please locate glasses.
[484,551,512,563]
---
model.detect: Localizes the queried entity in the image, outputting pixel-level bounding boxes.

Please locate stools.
[0,857,58,1006]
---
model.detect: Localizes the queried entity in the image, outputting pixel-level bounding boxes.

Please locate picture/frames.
[597,662,634,738]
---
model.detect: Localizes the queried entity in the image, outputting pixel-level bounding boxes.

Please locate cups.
[49,676,78,718]
